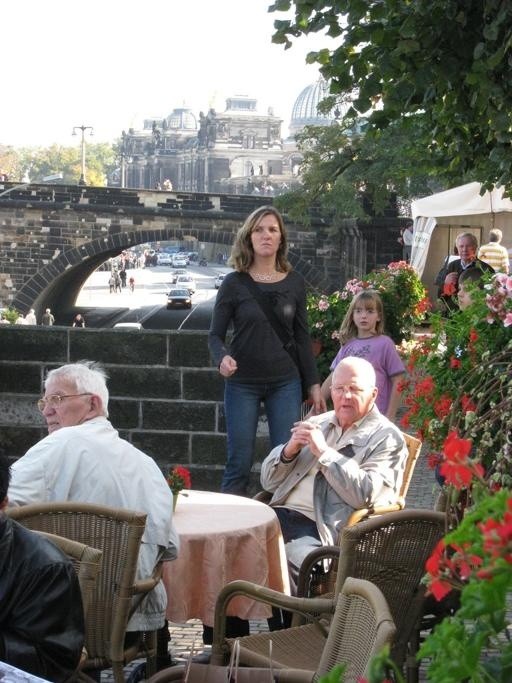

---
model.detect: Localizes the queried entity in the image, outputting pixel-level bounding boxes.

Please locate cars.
[166,289,192,310]
[172,255,187,268]
[176,275,196,293]
[172,269,187,284]
[157,254,172,265]
[113,322,143,329]
[214,273,227,289]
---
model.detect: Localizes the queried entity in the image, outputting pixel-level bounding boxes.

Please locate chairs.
[211,508,446,667]
[28,529,103,682]
[3,501,163,682]
[143,576,397,682]
[312,431,423,595]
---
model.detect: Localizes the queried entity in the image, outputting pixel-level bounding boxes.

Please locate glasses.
[37,392,92,412]
[353,308,374,314]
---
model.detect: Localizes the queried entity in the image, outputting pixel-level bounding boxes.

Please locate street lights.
[114,152,132,188]
[72,125,96,185]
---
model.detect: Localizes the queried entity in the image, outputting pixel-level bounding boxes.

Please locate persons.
[72,314,86,328]
[16,314,25,325]
[4,363,180,648]
[129,277,135,293]
[478,229,510,275]
[41,308,54,326]
[206,205,328,498]
[0,315,10,324]
[436,228,495,310]
[202,355,409,643]
[444,246,460,266]
[163,176,172,191]
[107,269,127,293]
[25,309,37,325]
[458,264,489,309]
[304,288,407,425]
[0,446,87,683]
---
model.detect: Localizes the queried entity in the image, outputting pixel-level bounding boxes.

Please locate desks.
[161,489,288,629]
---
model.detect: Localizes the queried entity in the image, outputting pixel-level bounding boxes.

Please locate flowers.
[164,467,191,495]
